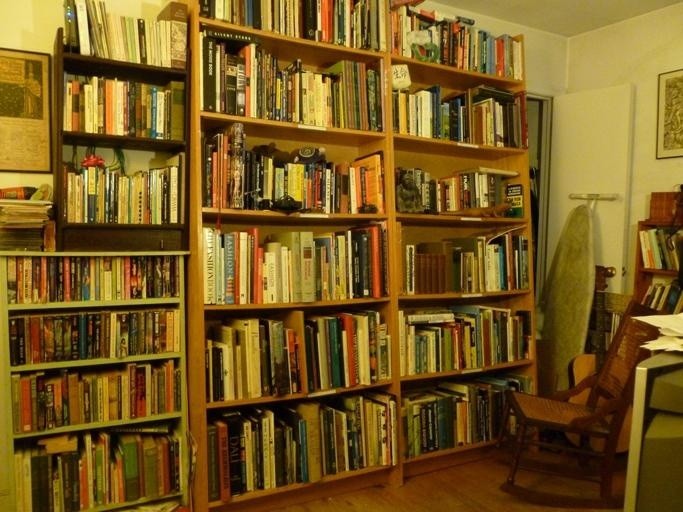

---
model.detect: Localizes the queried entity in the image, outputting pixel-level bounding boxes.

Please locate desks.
[622,341,682,512]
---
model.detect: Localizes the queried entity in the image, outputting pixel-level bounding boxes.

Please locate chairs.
[489,300,668,509]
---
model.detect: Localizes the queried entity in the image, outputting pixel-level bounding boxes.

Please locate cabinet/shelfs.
[194,45,397,511]
[52,22,191,252]
[394,44,537,489]
[0,256,190,512]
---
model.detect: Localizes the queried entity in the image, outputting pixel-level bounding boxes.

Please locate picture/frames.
[653,68,683,161]
[634,189,682,312]
[0,44,52,176]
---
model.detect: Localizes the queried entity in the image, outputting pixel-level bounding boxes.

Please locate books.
[197,1,399,502]
[389,2,530,459]
[637,225,683,315]
[1,1,188,512]
